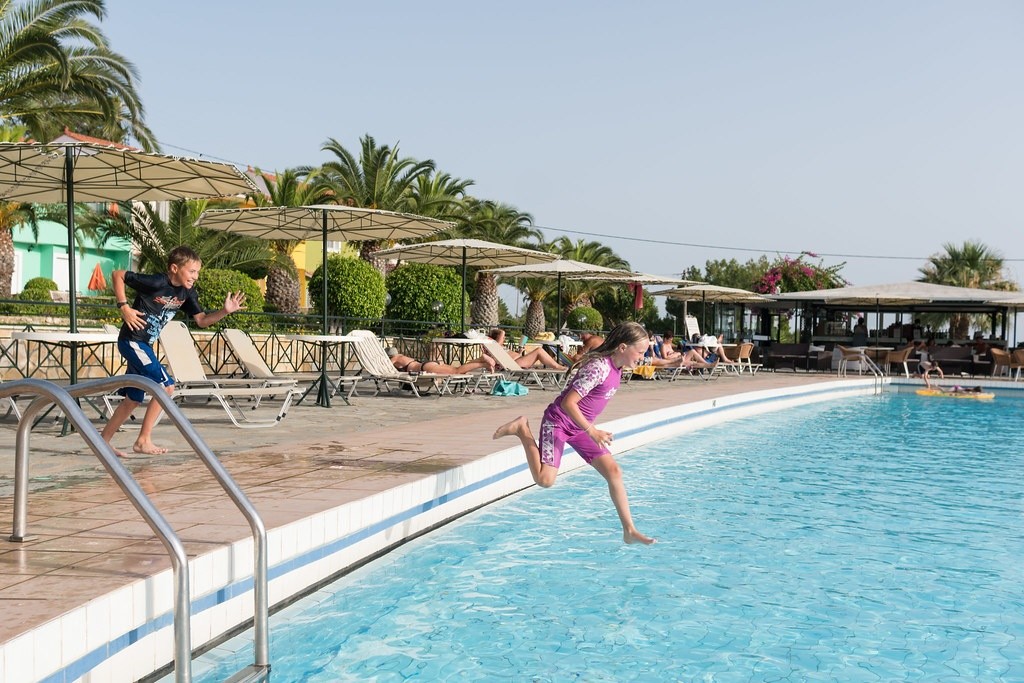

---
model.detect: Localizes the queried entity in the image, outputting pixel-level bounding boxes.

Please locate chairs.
[990,347,1013,378]
[222,327,362,410]
[1013,349,1024,382]
[157,321,308,430]
[478,338,565,389]
[343,328,424,399]
[836,343,868,379]
[545,342,764,382]
[885,346,914,378]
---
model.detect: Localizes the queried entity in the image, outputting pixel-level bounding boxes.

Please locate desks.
[431,336,486,395]
[859,345,895,377]
[12,331,119,436]
[285,333,362,409]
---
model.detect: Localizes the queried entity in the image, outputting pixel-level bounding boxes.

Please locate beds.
[360,370,473,399]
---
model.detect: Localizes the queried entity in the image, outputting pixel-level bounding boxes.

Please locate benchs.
[764,343,811,373]
[929,347,976,377]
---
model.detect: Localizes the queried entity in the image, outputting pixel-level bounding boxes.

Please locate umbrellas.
[828,281,936,373]
[0,131,776,359]
[980,288,1024,380]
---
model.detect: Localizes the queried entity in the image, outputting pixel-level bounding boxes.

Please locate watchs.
[117,302,129,309]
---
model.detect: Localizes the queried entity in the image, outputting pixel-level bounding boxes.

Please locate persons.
[691,332,734,364]
[384,347,496,375]
[648,330,665,359]
[644,329,684,367]
[99,245,248,459]
[853,318,869,337]
[660,332,721,370]
[491,321,656,547]
[572,330,686,368]
[904,335,989,395]
